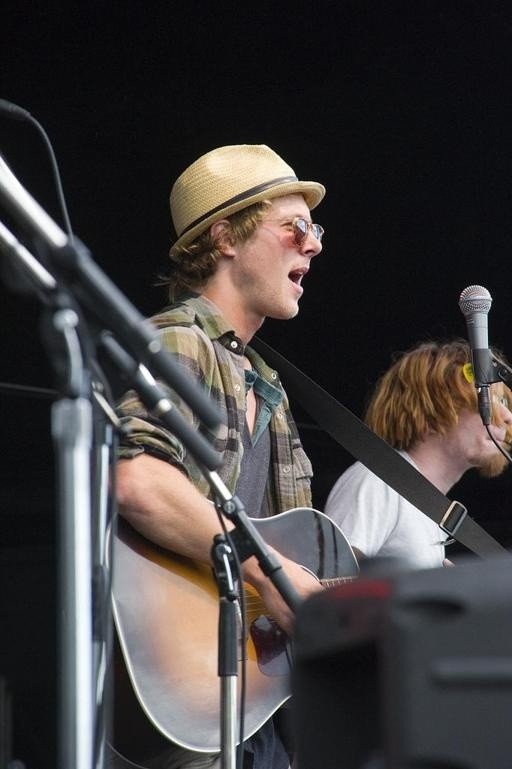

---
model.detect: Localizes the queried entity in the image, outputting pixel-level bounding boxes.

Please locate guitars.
[103,508,359,769]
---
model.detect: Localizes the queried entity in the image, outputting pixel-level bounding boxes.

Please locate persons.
[324,334,512,583]
[91,137,340,765]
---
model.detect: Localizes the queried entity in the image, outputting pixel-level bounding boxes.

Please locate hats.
[167,143,328,262]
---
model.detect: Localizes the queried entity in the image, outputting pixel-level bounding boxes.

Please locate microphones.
[458,285,493,425]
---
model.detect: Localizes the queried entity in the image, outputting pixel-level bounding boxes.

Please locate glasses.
[254,214,323,250]
